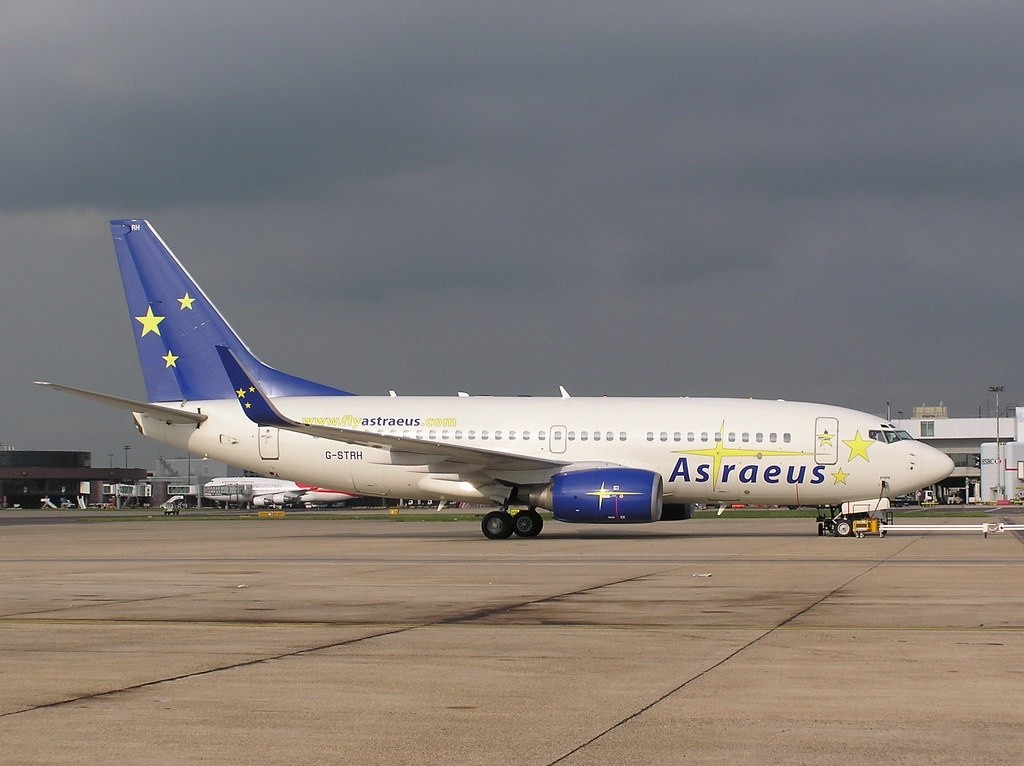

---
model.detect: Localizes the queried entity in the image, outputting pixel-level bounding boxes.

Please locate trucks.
[892,490,935,508]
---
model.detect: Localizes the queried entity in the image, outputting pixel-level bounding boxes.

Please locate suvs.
[165,502,180,515]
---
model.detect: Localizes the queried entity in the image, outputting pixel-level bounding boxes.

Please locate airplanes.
[32,217,954,536]
[200,473,357,510]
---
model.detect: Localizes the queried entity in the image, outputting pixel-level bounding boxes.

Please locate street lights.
[123,446,131,485]
[988,385,1006,500]
